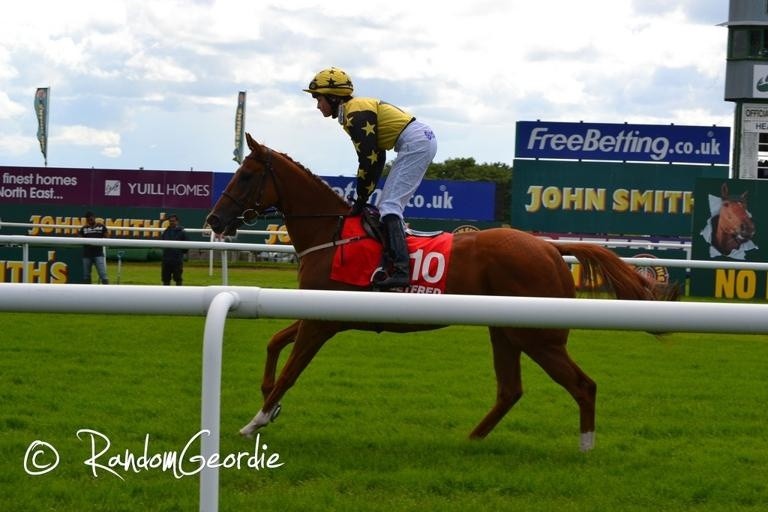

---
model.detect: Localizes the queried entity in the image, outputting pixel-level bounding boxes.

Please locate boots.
[373,213,412,289]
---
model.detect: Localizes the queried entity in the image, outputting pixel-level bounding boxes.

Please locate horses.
[714,181,754,255]
[205,131,683,452]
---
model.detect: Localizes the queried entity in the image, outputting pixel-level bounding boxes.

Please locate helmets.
[302,66,354,96]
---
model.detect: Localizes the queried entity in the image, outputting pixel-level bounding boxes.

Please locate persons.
[303,66,438,288]
[158,215,188,286]
[75,211,110,284]
